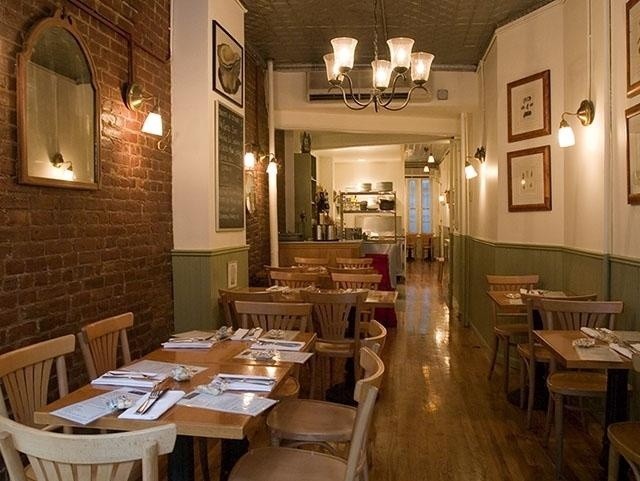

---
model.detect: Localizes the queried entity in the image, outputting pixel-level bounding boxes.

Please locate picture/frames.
[507,147,552,213]
[625,102,639,205]
[625,0,639,98]
[507,68,551,143]
[211,16,246,109]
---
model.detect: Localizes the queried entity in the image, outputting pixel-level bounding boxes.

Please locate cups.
[313,224,336,241]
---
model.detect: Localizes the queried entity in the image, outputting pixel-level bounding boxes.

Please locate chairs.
[406,230,447,284]
[0,312,386,481]
[217,256,397,399]
[485,273,639,480]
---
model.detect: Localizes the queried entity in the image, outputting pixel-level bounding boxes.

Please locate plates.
[94,370,167,388]
[119,390,185,421]
[504,292,521,299]
[363,184,371,192]
[575,339,596,349]
[233,328,263,342]
[209,374,274,394]
[377,182,393,192]
[164,343,214,350]
[251,339,306,352]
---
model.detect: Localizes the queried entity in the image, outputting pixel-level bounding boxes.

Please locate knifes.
[143,387,170,416]
[241,327,252,339]
[102,376,162,383]
[219,377,275,382]
[255,340,301,347]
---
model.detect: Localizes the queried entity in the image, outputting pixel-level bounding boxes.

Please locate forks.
[137,385,163,414]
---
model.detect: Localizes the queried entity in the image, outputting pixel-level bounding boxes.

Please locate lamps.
[128,82,166,139]
[423,147,429,173]
[321,0,438,114]
[43,148,80,182]
[246,140,281,177]
[439,190,449,206]
[464,147,490,180]
[428,144,435,164]
[557,98,596,148]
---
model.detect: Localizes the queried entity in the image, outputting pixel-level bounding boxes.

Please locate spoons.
[249,328,260,336]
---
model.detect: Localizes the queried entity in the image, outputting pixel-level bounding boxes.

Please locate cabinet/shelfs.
[340,192,397,246]
[294,152,318,241]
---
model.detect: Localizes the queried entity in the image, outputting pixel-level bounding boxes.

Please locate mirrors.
[14,10,104,191]
